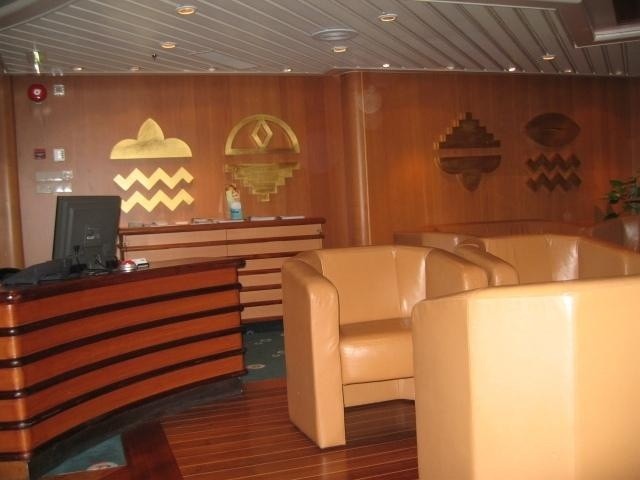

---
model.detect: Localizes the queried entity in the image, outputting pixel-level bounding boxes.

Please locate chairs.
[410,273,640,480]
[281,246,491,450]
[393,218,584,251]
[577,215,639,254]
[452,234,639,288]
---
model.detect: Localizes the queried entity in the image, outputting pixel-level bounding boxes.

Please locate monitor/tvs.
[52,195,121,276]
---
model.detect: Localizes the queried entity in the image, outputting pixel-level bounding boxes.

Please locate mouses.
[119,260,137,270]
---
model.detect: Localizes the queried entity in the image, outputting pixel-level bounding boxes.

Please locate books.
[148,216,305,226]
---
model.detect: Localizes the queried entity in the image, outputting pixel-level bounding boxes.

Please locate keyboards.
[3,260,55,286]
